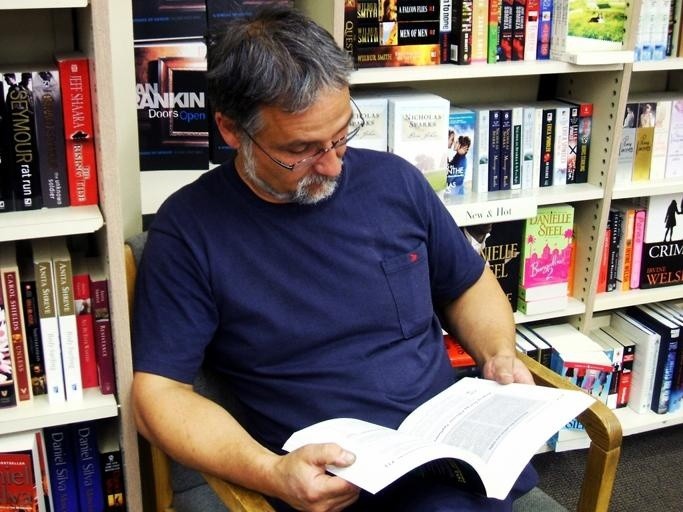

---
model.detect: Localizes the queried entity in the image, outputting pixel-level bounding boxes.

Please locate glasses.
[234,96,366,172]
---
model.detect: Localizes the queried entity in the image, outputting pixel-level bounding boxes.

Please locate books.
[134,1,293,168]
[345,1,683,453]
[0,1,125,512]
[280,376,597,507]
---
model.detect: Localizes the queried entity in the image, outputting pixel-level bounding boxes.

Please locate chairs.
[122,230,624,511]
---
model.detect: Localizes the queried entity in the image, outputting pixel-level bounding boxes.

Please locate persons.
[130,5,539,512]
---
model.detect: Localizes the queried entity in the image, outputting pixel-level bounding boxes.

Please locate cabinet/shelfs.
[0,0,682,511]
